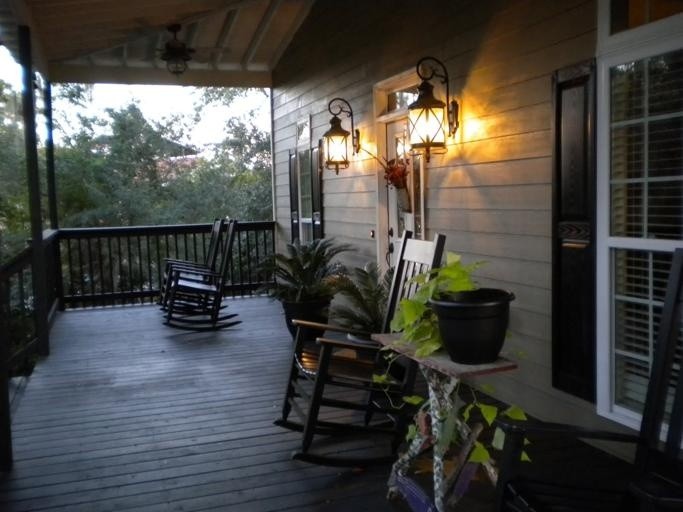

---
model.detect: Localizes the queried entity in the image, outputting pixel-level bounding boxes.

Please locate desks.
[367,326,520,511]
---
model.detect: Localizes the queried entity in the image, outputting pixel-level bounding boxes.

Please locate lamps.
[406,58,459,163]
[320,98,359,174]
[160,23,198,77]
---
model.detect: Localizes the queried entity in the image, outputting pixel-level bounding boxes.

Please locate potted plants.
[393,250,536,480]
[242,236,363,341]
[318,259,395,358]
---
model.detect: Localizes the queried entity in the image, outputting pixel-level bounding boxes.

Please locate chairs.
[274,229,447,469]
[495,247,682,512]
[160,217,241,330]
[159,217,227,312]
